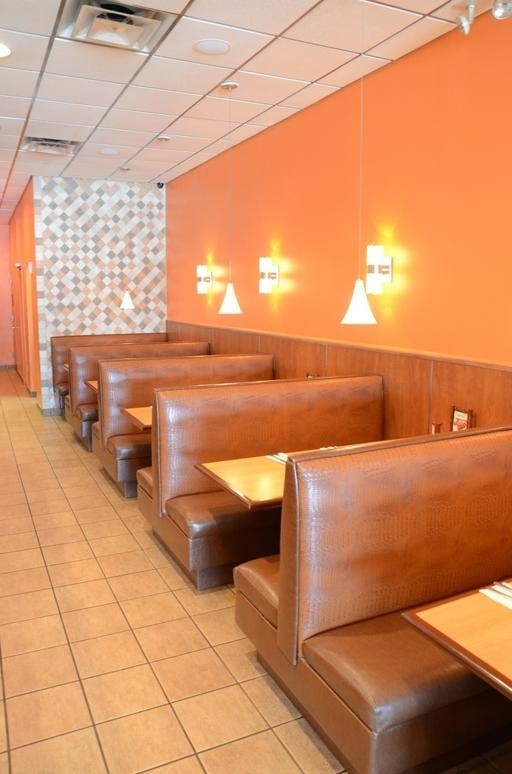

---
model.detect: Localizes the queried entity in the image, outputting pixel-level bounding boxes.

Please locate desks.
[399,577,512,708]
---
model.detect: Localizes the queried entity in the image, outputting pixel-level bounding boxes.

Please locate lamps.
[258,257,279,292]
[365,243,392,295]
[217,82,243,316]
[339,52,377,326]
[456,0,512,37]
[197,264,213,293]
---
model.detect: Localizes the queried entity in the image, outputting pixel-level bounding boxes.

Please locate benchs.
[233,424,511,773]
[51,329,382,593]
